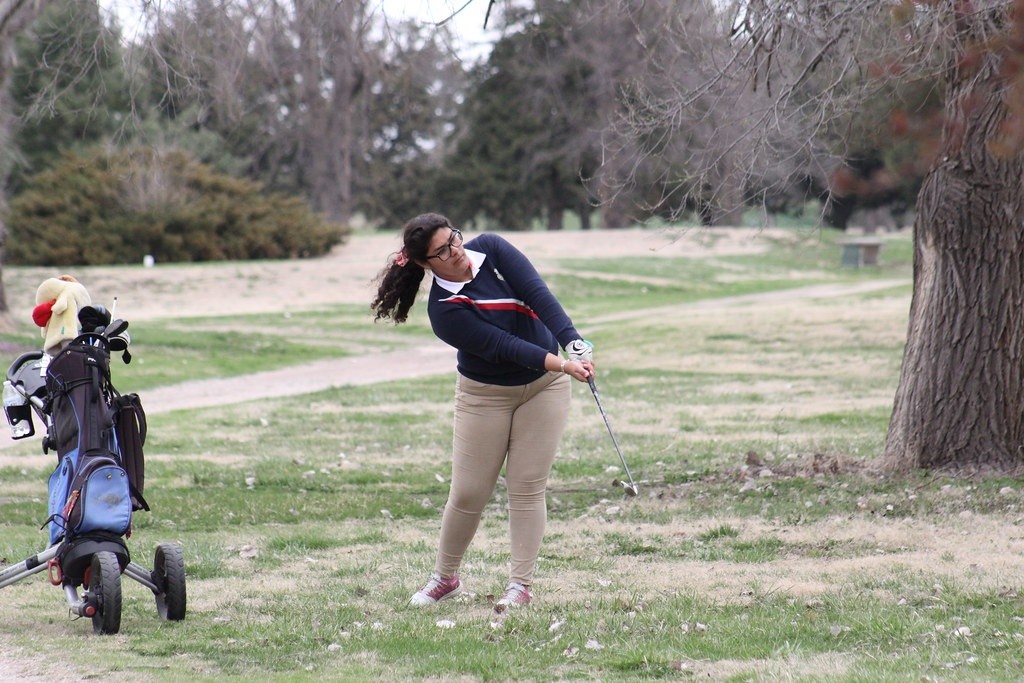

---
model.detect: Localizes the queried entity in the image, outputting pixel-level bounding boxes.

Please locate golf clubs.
[78,296,132,365]
[587,376,638,497]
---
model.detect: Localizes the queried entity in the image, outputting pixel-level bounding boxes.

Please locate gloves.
[566,340,592,363]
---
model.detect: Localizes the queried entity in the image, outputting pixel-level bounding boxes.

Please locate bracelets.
[559,357,571,376]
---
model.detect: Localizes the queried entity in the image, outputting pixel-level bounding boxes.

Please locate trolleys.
[0,349,188,638]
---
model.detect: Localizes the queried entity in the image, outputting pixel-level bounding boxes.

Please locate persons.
[367,211,596,616]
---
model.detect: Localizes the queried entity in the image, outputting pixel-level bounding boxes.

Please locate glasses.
[419,229,463,261]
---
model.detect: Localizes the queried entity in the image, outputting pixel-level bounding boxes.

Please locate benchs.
[834,236,885,269]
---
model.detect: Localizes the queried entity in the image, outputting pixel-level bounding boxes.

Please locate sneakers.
[493,585,532,609]
[410,573,463,610]
